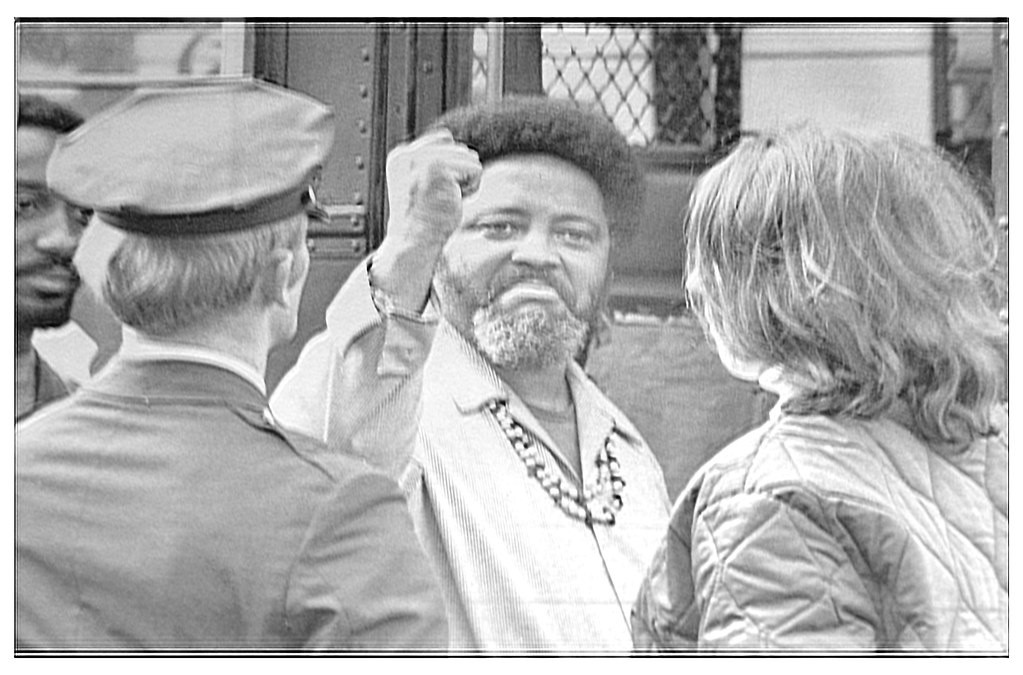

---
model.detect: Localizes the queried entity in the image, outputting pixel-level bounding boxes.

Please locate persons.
[260,97,673,654]
[627,113,1008,656]
[17,91,96,426]
[14,75,455,655]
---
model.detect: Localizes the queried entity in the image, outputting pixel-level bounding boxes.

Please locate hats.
[45,76,339,237]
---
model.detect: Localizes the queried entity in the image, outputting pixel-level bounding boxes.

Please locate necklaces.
[483,395,627,529]
